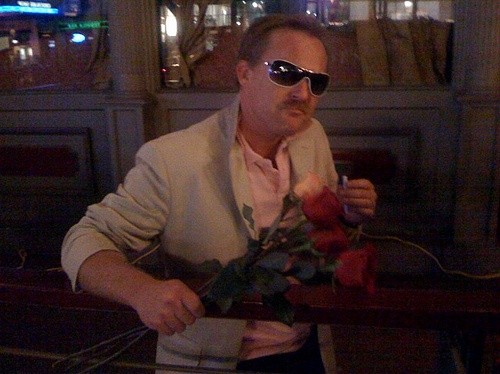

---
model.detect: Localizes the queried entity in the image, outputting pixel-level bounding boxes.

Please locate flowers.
[51,181,378,374]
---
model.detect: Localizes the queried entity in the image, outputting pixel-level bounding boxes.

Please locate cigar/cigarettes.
[341,175,349,214]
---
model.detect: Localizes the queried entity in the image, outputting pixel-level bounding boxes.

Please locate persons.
[61,15,377,373]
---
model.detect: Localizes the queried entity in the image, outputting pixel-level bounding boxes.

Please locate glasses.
[242,54,331,97]
